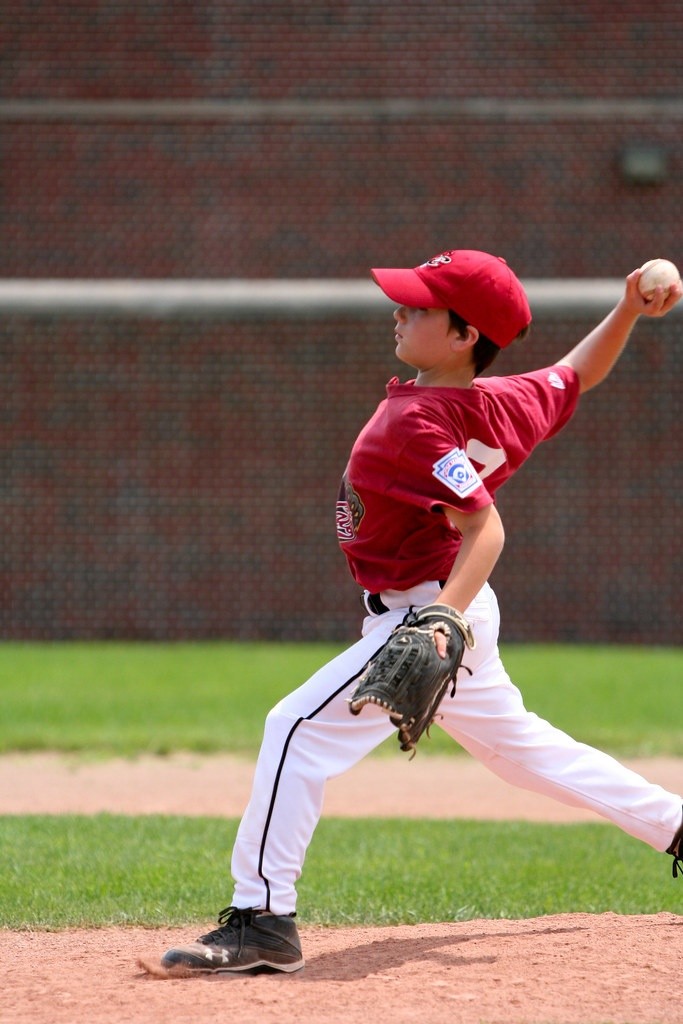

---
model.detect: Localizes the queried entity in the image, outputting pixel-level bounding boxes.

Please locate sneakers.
[666,822,683,879]
[161,904,306,974]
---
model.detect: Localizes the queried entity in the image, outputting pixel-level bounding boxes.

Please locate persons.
[159,249,683,975]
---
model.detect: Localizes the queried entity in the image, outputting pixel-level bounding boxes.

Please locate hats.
[370,250,533,350]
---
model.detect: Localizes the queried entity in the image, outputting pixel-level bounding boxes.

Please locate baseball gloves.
[341,601,478,763]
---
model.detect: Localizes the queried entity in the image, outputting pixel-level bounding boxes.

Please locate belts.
[360,577,446,616]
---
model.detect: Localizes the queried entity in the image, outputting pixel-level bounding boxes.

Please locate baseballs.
[636,257,681,302]
